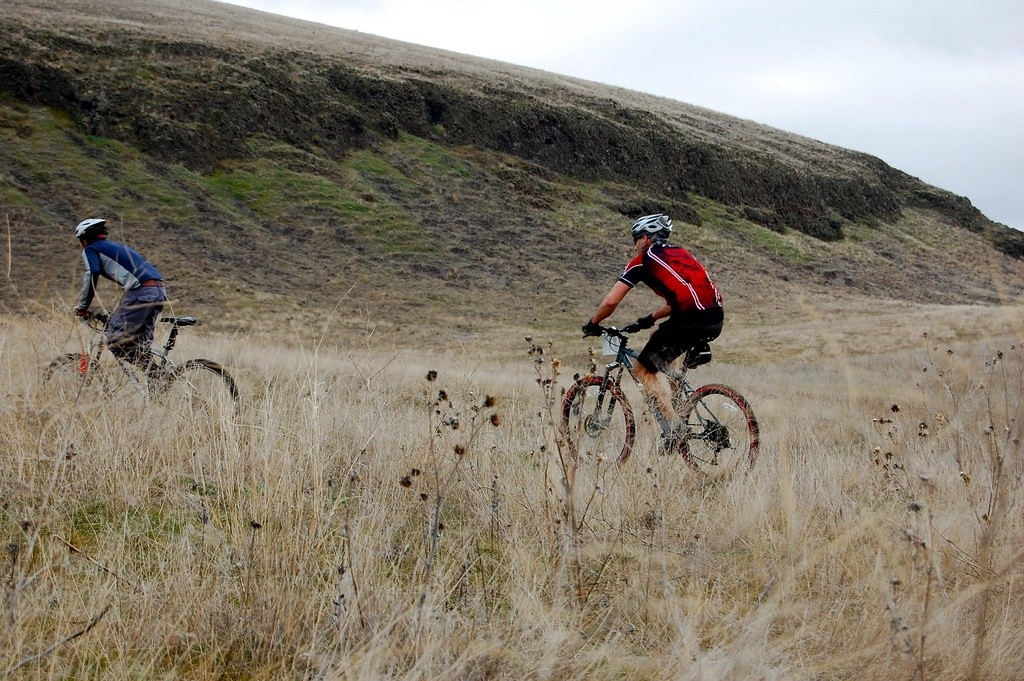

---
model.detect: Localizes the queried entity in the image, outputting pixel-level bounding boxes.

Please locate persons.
[580,213,724,455]
[72,218,174,403]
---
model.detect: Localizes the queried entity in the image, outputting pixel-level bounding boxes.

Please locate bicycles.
[559,319,762,487]
[39,305,242,429]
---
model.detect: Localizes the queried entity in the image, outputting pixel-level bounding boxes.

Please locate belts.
[142,280,165,287]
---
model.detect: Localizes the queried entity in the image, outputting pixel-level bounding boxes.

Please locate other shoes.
[669,395,684,412]
[657,422,692,456]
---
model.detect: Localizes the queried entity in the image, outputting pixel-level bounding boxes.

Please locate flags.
[80,353,86,372]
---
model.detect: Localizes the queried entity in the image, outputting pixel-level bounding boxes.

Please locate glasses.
[633,238,639,245]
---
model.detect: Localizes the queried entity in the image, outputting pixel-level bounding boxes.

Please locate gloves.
[73,304,87,316]
[582,318,599,336]
[636,313,657,329]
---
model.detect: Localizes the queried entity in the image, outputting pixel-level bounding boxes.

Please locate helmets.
[76,218,106,239]
[631,214,672,236]
